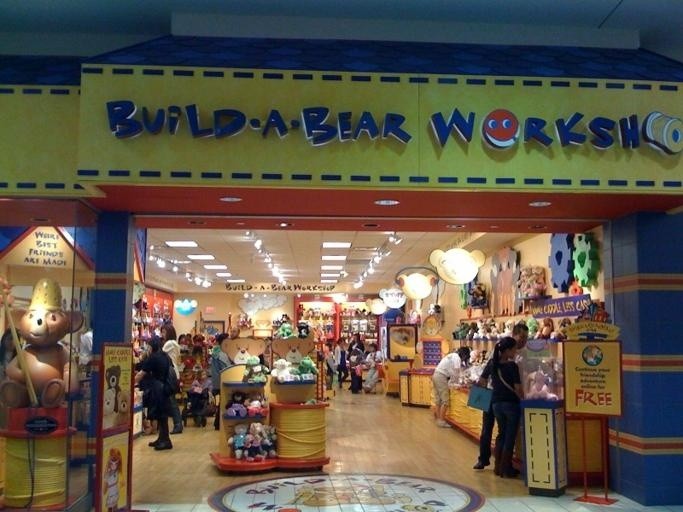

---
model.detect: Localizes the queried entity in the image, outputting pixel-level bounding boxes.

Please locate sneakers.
[437,420,452,428]
[494,467,520,479]
[148,426,184,451]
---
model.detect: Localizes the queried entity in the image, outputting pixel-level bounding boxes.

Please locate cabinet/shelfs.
[132,286,175,358]
[294,295,381,347]
[210,363,330,471]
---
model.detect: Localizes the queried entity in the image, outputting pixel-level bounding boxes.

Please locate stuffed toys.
[524,266,548,314]
[467,349,559,400]
[467,282,486,305]
[451,317,571,340]
[273,321,333,340]
[516,266,532,312]
[303,303,371,320]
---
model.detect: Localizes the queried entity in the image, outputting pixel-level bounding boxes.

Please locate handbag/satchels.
[165,366,181,397]
[466,384,495,414]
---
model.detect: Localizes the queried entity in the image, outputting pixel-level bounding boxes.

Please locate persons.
[135,336,174,450]
[186,380,209,428]
[472,324,529,470]
[204,334,231,430]
[325,333,382,394]
[0,327,23,380]
[479,335,524,477]
[161,324,184,435]
[431,346,471,428]
[78,321,94,376]
[230,328,240,339]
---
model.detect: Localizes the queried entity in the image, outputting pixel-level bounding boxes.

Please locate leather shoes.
[473,458,490,469]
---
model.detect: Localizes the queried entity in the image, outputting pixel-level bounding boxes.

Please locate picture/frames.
[206,321,224,335]
